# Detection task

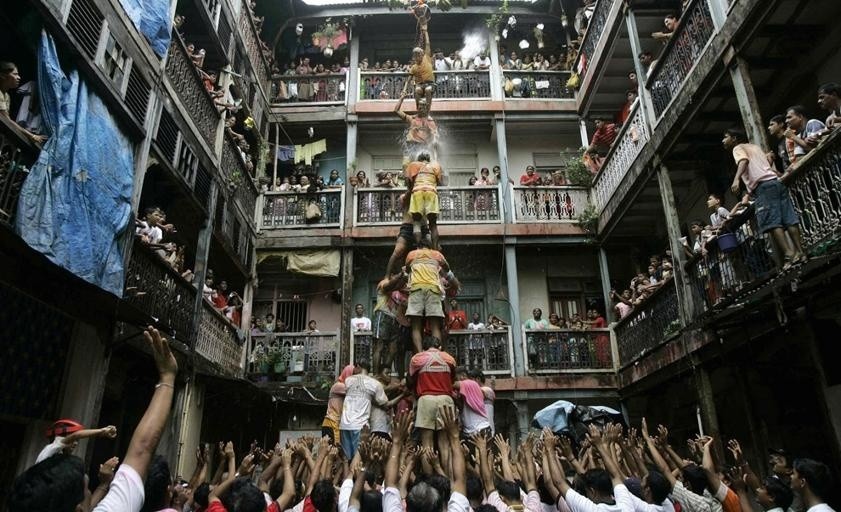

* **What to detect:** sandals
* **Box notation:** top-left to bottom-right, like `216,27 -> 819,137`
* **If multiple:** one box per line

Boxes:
779,252 -> 807,271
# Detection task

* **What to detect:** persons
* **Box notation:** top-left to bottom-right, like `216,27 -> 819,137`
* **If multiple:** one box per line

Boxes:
243,1 -> 416,108
467,1 -> 707,221
2,63 -> 50,148
174,12 -> 408,224
302,320 -> 321,372
2,324 -> 840,512
125,206 -> 242,341
609,86 -> 839,366
433,2 -> 609,98
370,1 -> 464,386
250,313 -> 292,372
324,304 -> 372,373
467,303 -> 614,380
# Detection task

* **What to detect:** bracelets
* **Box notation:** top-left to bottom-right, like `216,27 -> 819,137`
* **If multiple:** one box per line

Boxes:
360,467 -> 366,471
155,383 -> 174,389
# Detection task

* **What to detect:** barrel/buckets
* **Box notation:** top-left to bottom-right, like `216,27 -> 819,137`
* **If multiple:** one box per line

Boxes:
716,224 -> 738,253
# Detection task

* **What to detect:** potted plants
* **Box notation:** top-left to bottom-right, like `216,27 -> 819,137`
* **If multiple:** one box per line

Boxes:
348,157 -> 359,187
255,353 -> 269,371
533,25 -> 543,49
311,17 -> 349,57
269,347 -> 286,373
294,354 -> 304,371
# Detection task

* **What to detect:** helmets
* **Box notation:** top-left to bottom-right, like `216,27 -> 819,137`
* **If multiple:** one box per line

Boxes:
47,420 -> 83,437
